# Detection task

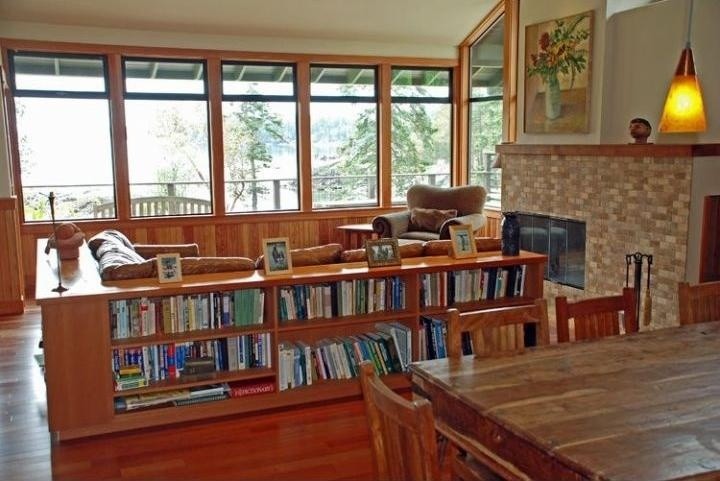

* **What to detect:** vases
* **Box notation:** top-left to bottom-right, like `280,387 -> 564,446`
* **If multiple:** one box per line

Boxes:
502,211 -> 520,256
546,74 -> 562,119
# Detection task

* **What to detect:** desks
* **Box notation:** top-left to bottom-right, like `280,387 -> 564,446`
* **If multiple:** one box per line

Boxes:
408,321 -> 720,481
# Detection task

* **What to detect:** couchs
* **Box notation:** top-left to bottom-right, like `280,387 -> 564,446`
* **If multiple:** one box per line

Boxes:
372,185 -> 486,239
88,228 -> 501,281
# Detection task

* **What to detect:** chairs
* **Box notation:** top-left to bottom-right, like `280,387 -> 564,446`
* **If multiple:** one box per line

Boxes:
679,282 -> 720,324
555,288 -> 637,343
441,298 -> 550,481
360,360 -> 440,481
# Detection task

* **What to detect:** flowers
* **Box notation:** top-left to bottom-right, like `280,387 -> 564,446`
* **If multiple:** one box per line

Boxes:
526,16 -> 590,89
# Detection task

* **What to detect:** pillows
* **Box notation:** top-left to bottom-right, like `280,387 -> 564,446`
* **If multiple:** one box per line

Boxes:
408,208 -> 458,232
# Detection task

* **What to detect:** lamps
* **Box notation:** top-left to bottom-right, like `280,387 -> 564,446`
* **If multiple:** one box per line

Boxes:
657,0 -> 707,134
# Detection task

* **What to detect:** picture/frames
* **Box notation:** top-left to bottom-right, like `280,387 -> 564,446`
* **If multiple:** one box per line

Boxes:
366,238 -> 401,268
156,252 -> 181,284
262,236 -> 292,275
524,9 -> 595,135
448,225 -> 478,259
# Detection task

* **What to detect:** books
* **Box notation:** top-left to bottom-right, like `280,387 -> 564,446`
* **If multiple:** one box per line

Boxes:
279,321 -> 413,391
109,288 -> 265,341
278,276 -> 406,321
115,379 -> 273,412
418,314 -> 475,362
418,264 -> 526,308
112,332 -> 272,391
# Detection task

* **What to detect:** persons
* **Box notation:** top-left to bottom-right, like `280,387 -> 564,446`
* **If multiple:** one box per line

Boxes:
628,118 -> 655,146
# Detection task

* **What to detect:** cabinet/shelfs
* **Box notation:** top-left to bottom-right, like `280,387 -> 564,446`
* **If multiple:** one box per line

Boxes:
35,237 -> 549,441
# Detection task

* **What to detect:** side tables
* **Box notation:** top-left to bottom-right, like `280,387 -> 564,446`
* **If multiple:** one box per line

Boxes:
336,223 -> 373,249
